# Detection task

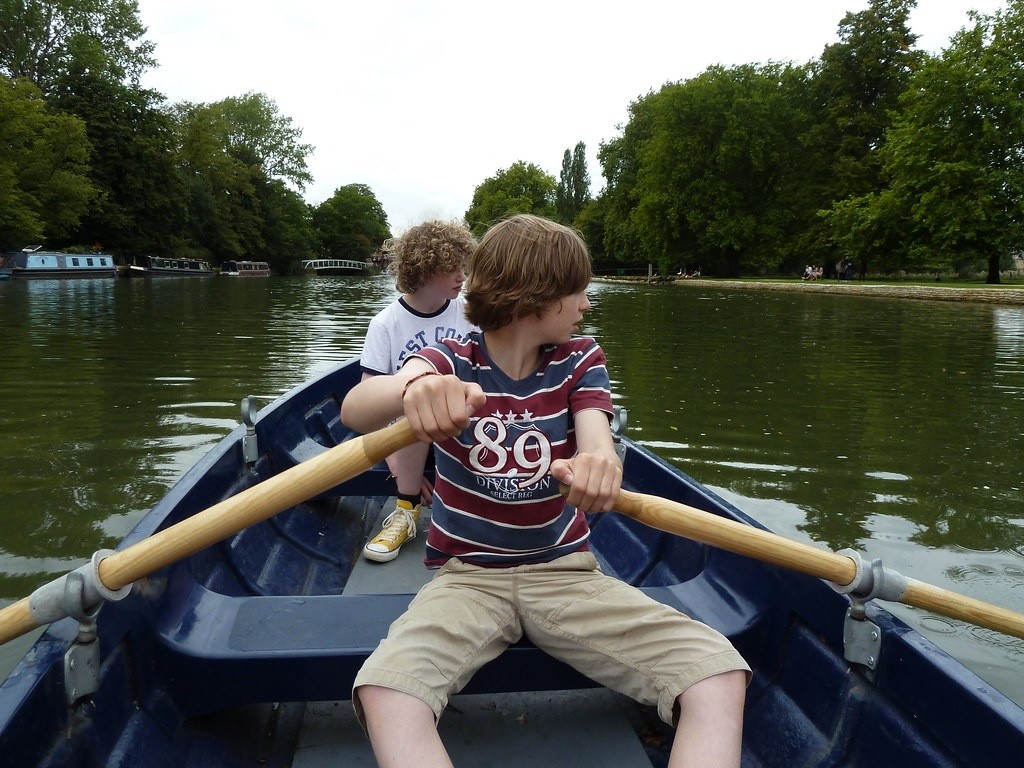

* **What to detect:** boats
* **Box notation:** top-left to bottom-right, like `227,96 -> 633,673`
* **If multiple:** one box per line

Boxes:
11,245 -> 117,277
216,260 -> 272,278
0,356 -> 1024,768
127,254 -> 213,276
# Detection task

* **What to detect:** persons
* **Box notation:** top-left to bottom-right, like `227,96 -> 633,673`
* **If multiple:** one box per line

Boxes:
676,268 -> 700,278
801,253 -> 868,281
341,213 -> 752,768
358,217 -> 478,563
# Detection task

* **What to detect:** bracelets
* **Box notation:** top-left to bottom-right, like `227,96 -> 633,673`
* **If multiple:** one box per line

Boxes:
402,371 -> 442,402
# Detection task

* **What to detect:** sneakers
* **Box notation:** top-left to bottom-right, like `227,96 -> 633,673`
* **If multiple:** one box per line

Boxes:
363,497 -> 423,562
